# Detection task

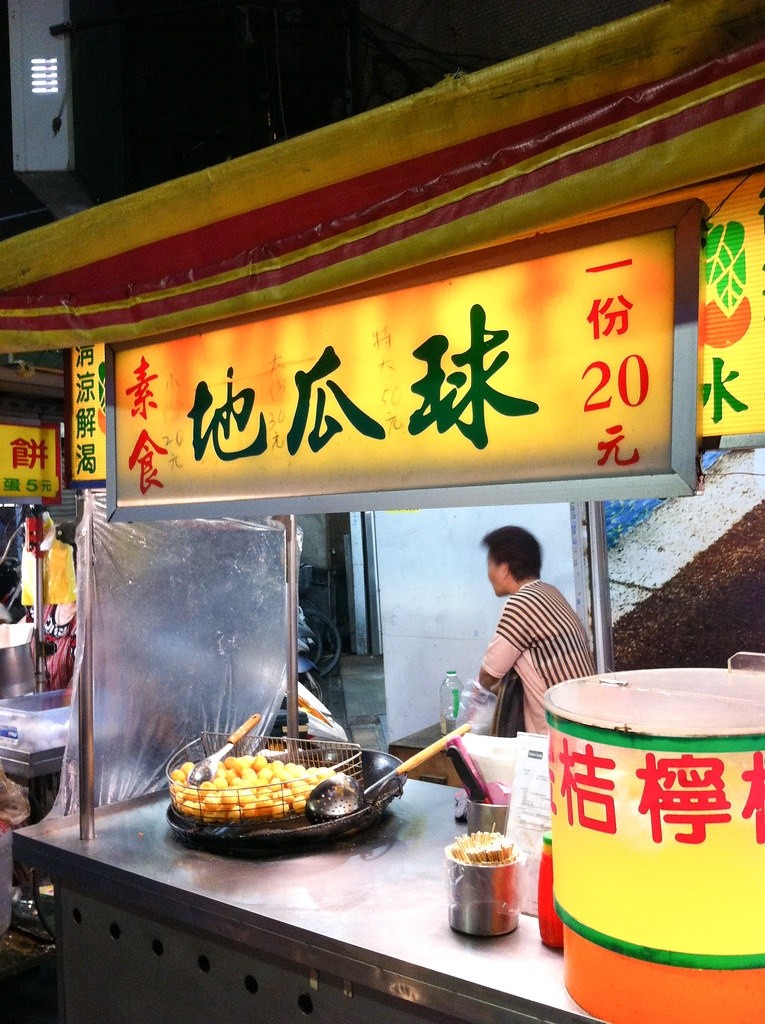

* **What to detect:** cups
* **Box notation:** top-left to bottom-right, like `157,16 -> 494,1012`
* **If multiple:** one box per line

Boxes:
443,847 -> 522,936
466,797 -> 508,836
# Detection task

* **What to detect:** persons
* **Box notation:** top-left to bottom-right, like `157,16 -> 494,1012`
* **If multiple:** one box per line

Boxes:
479,526 -> 596,735
0,564 -> 19,678
17,599 -> 76,708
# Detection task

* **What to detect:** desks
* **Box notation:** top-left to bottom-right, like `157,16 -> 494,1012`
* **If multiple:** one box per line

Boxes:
388,721 -> 461,787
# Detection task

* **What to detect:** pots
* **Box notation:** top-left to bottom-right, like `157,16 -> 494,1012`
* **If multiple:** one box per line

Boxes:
163,747 -> 407,854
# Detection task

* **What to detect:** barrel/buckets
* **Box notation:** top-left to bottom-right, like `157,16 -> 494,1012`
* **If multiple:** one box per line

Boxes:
0,643 -> 37,698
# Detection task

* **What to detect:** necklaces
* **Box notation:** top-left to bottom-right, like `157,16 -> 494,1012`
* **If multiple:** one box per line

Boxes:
55,607 -> 76,627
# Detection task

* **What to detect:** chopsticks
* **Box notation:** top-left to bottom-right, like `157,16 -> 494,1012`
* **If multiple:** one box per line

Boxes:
451,822 -> 516,864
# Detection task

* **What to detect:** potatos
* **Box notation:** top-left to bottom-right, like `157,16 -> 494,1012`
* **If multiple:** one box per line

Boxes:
170,755 -> 340,823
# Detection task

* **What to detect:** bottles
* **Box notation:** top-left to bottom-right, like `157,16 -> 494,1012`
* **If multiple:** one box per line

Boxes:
438,670 -> 464,736
536,831 -> 564,948
